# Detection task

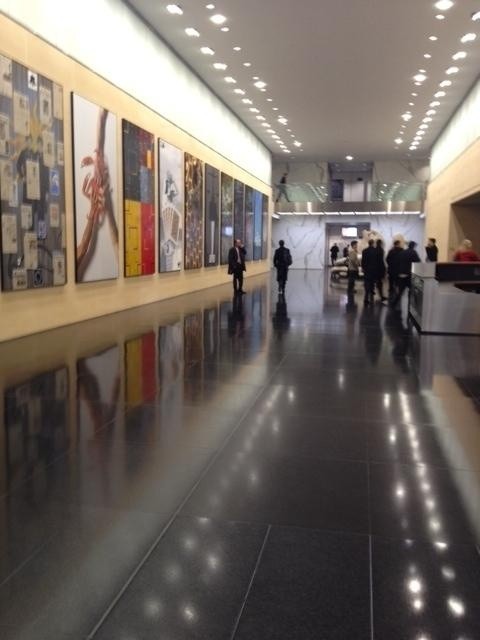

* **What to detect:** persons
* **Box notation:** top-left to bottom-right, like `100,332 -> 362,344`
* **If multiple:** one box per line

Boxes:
228,238 -> 247,296
453,239 -> 480,261
330,236 -> 438,307
274,239 -> 292,289
80,156 -> 118,249
276,172 -> 291,203
